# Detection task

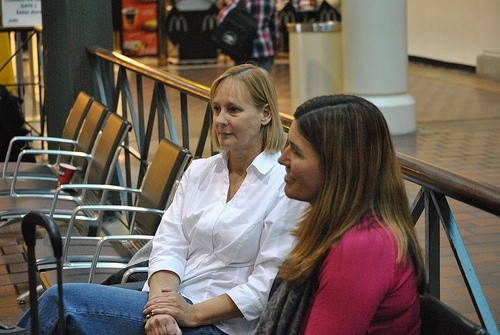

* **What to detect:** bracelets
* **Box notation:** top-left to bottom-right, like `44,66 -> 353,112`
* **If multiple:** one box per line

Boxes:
146,311 -> 156,320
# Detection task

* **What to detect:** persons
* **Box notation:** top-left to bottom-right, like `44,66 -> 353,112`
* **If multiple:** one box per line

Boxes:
217,0 -> 280,74
253,92 -> 423,335
18,61 -> 311,335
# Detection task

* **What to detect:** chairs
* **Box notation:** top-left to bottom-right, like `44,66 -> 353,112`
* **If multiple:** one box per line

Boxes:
0,91 -> 491,335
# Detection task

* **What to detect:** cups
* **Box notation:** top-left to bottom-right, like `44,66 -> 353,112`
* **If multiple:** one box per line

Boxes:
57,162 -> 77,186
